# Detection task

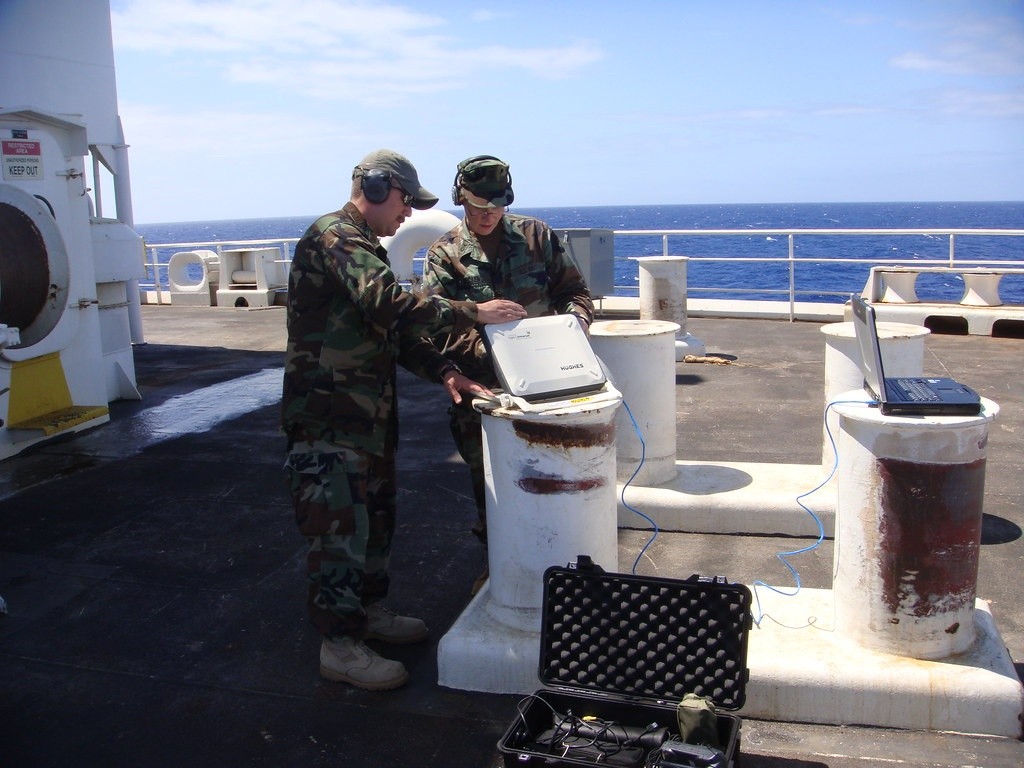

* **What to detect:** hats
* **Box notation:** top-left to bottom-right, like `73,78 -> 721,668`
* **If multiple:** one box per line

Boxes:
353,155 -> 439,210
457,157 -> 514,209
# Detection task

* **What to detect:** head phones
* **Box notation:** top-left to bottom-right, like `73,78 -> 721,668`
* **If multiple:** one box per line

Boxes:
361,168 -> 393,204
451,155 -> 512,206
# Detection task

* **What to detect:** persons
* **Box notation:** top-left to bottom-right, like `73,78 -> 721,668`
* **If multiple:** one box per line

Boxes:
279,149 -> 528,692
422,154 -> 595,597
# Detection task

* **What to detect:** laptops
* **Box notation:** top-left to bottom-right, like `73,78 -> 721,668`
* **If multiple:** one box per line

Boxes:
850,293 -> 983,417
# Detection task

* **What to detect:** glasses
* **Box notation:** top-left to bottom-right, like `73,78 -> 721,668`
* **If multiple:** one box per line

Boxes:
391,186 -> 416,206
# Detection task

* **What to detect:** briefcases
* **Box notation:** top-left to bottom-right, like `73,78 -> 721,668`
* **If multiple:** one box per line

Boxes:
496,554 -> 752,768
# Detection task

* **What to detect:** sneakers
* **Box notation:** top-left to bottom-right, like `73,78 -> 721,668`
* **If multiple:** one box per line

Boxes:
319,635 -> 408,691
362,609 -> 429,644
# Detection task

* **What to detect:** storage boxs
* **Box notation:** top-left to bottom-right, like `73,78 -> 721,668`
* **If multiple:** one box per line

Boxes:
495,555 -> 754,768
